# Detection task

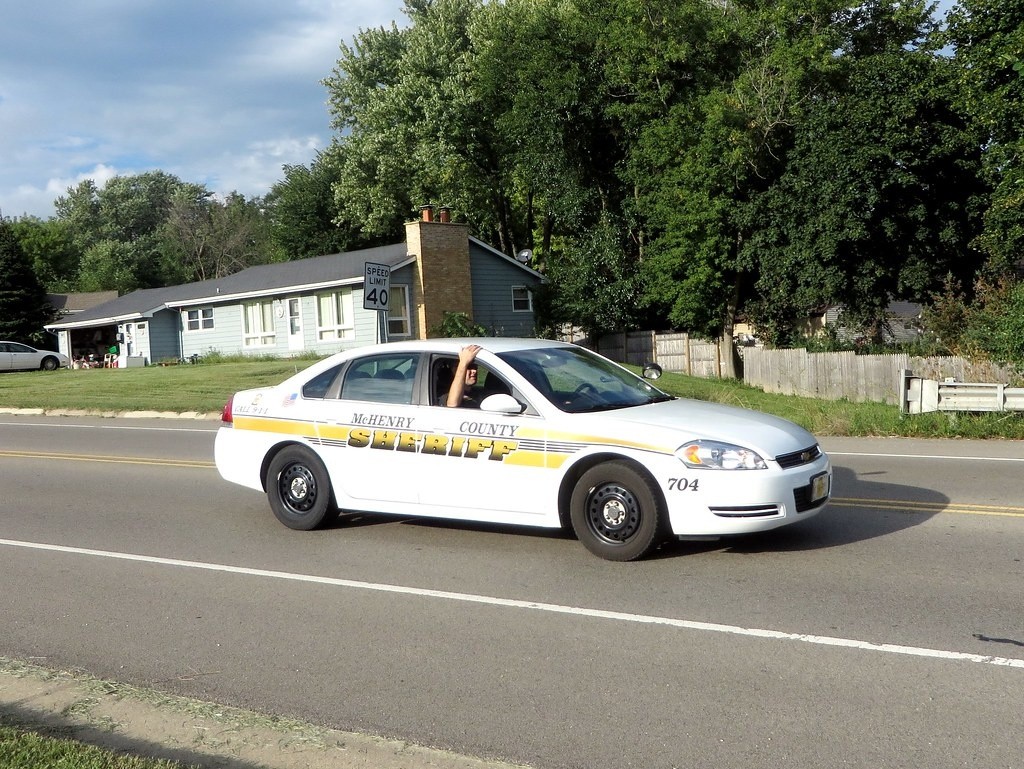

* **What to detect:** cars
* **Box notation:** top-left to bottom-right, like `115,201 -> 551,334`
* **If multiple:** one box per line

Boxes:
0,340 -> 69,371
214,338 -> 834,562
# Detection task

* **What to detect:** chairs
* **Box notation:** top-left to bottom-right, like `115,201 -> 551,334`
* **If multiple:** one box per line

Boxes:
483,371 -> 511,397
103,354 -> 113,368
373,369 -> 410,404
344,371 -> 383,403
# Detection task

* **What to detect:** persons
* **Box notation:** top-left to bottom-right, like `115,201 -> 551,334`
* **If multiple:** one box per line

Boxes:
441,344 -> 483,408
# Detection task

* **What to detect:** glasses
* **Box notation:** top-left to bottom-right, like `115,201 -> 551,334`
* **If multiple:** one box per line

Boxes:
453,363 -> 478,370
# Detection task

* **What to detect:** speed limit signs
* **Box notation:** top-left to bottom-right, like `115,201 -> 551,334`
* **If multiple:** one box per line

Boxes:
362,262 -> 390,311
